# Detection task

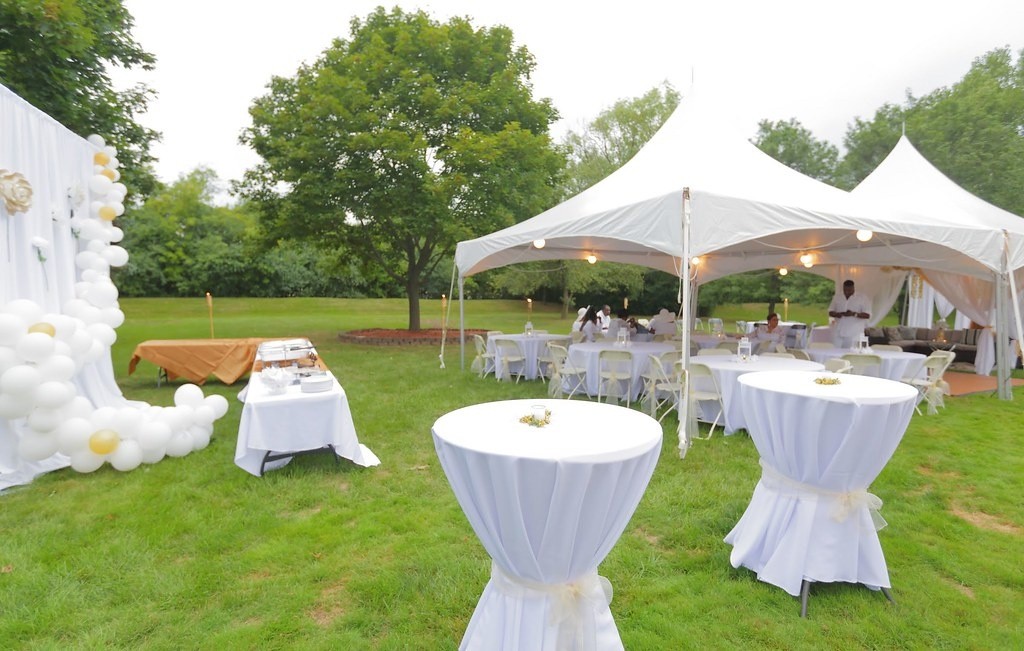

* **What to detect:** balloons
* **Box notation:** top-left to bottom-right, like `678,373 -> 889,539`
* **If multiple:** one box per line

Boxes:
0,134 -> 229,472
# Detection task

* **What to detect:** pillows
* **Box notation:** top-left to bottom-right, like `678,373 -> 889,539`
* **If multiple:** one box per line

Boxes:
864,327 -> 982,345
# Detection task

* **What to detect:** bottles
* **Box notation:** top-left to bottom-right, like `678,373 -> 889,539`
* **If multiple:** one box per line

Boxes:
525,322 -> 533,336
738,337 -> 751,358
617,328 -> 630,345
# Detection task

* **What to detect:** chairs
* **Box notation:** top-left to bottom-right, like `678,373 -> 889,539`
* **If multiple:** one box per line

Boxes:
472,320 -> 956,440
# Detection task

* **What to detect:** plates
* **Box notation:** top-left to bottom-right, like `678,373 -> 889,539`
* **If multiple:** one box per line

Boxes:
300,375 -> 333,393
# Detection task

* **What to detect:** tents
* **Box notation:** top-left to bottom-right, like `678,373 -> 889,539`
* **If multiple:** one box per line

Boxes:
456,77 -> 1024,454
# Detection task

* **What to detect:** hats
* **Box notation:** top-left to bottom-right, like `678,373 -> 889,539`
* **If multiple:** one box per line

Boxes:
654,309 -> 676,323
576,305 -> 591,322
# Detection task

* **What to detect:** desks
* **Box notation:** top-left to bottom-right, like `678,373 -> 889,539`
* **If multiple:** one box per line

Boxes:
723,371 -> 919,619
811,326 -> 834,342
634,331 -> 720,350
801,346 -> 926,382
673,356 -> 827,436
234,338 -> 380,478
668,334 -> 776,356
749,321 -> 808,348
564,341 -> 676,401
127,338 -> 303,388
488,335 -> 572,381
433,400 -> 663,651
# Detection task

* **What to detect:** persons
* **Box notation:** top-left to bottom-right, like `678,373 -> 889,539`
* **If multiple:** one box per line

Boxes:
649,309 -> 677,335
736,313 -> 786,352
828,280 -> 870,350
606,308 -> 638,340
579,305 -> 603,342
596,304 -> 611,329
572,308 -> 586,331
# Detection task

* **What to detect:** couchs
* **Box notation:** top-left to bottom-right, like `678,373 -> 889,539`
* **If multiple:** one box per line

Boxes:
860,326 -> 999,368
